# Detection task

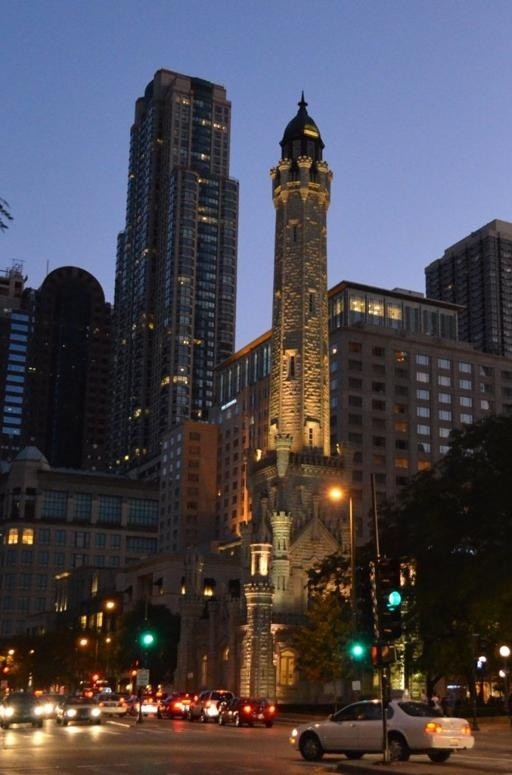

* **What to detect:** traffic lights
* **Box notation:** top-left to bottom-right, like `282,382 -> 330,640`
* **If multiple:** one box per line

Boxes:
371,645 -> 396,668
353,642 -> 364,666
135,625 -> 141,668
142,624 -> 154,668
356,560 -> 378,646
378,557 -> 402,640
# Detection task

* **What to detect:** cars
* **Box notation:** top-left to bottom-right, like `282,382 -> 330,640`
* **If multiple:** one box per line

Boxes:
288,699 -> 474,763
1,689 -> 276,729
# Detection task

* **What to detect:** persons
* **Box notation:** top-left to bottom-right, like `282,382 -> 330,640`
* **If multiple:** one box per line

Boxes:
420,687 -> 427,705
401,687 -> 411,701
428,696 -> 444,716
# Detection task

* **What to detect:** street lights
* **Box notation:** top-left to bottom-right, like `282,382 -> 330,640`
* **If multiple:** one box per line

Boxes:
329,487 -> 359,705
499,645 -> 511,712
